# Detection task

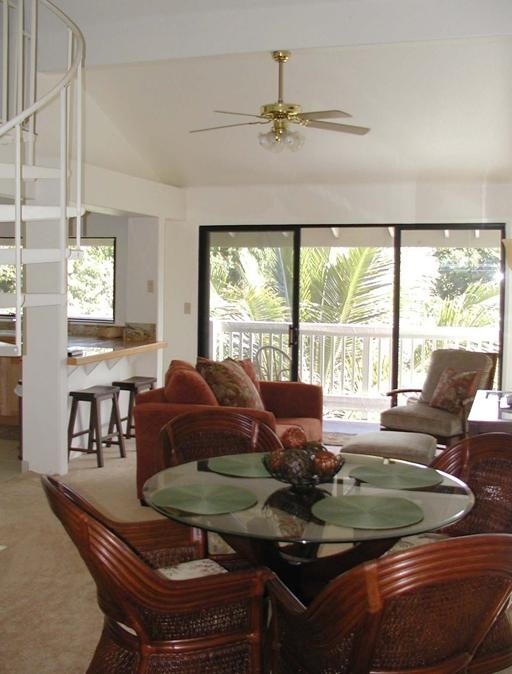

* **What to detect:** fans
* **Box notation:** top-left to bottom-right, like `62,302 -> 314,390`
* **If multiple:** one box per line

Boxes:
188,50 -> 375,138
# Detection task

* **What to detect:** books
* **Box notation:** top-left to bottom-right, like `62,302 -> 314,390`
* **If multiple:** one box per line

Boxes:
69,349 -> 84,357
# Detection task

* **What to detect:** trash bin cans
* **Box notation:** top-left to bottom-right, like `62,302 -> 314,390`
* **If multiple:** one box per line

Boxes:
14,379 -> 22,461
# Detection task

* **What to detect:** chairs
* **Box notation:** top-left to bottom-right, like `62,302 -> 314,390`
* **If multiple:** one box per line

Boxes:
377,348 -> 499,458
158,410 -> 284,469
254,345 -> 303,382
258,533 -> 512,674
430,432 -> 511,535
41,472 -> 271,672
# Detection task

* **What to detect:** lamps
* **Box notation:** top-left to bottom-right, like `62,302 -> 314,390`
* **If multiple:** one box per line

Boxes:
255,119 -> 304,151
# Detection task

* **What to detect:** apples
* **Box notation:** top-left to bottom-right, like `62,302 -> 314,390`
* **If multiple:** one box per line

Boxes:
270,428 -> 337,480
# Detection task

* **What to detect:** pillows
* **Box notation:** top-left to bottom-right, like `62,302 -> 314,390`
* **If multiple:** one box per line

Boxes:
426,364 -> 476,415
164,354 -> 266,410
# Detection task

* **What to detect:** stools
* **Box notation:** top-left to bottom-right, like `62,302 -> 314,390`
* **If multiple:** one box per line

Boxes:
103,377 -> 157,445
64,384 -> 127,468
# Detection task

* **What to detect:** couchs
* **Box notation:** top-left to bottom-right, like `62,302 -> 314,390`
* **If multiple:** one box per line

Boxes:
132,380 -> 325,511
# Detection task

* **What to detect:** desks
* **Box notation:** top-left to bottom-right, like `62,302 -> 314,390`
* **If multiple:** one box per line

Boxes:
143,448 -> 476,608
466,387 -> 512,440
